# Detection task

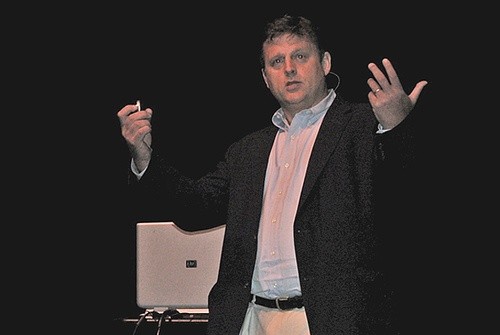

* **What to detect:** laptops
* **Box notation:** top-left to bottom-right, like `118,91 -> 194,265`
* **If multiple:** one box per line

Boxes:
137,221 -> 227,319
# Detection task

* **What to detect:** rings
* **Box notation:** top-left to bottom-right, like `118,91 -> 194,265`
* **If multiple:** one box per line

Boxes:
374,88 -> 381,94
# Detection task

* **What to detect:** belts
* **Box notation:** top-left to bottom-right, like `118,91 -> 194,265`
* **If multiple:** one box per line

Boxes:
249,294 -> 304,311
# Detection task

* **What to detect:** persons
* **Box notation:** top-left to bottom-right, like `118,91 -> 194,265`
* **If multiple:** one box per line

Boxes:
117,13 -> 443,335
